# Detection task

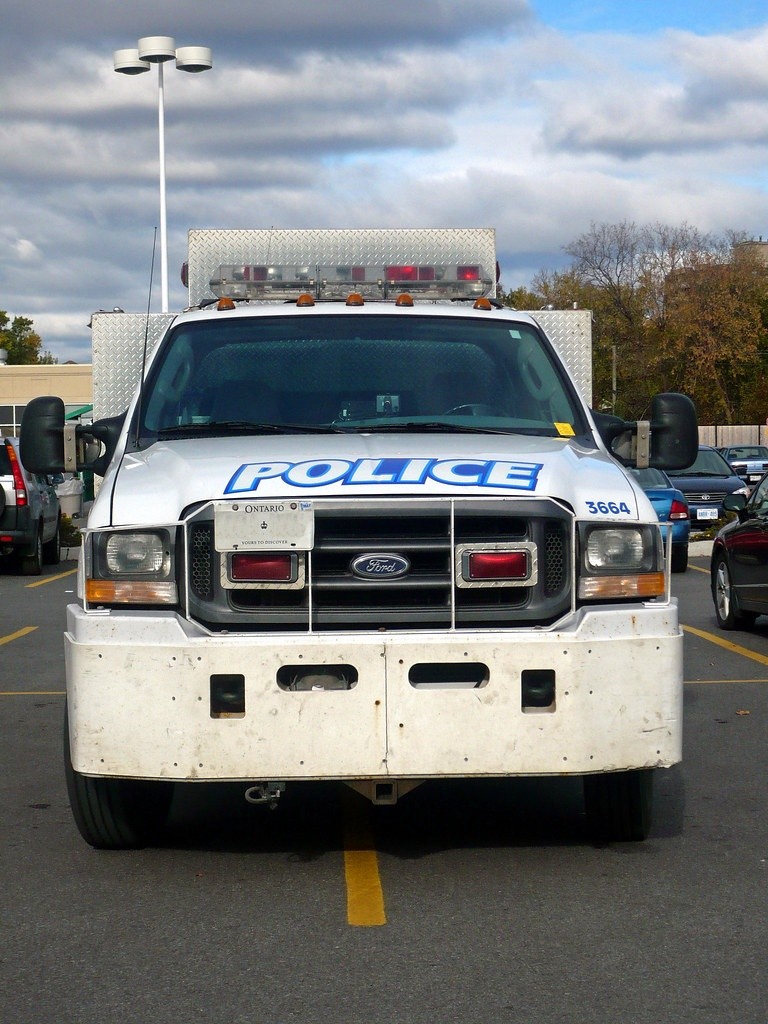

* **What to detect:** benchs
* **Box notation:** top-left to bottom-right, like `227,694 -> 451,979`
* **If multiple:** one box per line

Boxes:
278,386 -> 420,422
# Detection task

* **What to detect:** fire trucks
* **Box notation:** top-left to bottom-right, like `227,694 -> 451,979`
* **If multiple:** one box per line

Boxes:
20,223 -> 707,847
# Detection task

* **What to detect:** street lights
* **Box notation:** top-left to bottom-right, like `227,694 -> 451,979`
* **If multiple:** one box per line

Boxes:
110,34 -> 211,314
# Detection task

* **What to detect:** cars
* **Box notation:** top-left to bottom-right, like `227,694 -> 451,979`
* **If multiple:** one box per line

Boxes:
709,466 -> 768,629
661,444 -> 752,528
720,445 -> 768,483
628,465 -> 690,573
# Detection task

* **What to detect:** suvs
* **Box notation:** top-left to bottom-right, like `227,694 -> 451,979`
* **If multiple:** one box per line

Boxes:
0,436 -> 65,577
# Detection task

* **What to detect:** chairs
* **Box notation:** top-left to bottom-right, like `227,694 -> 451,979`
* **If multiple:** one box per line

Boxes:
424,371 -> 472,419
206,378 -> 280,424
